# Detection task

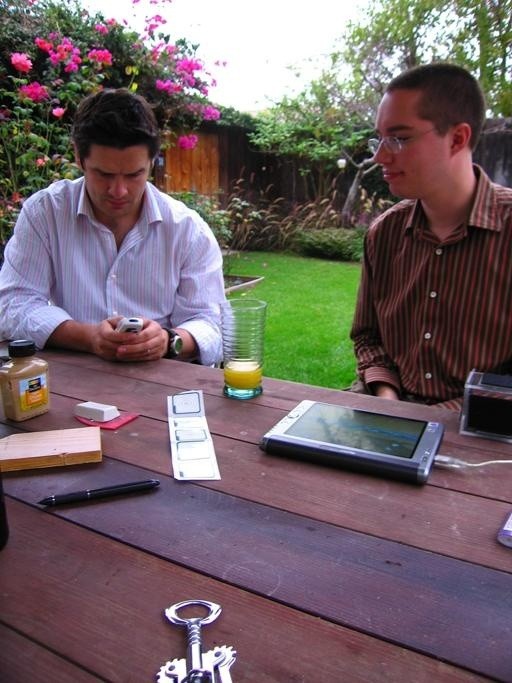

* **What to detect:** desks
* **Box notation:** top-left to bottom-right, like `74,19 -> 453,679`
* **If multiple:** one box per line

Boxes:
0,340 -> 512,683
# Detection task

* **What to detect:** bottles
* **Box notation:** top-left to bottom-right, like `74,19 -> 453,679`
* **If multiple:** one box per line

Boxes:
0,339 -> 51,423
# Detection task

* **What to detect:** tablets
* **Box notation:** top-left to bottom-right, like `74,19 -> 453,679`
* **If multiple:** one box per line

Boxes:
256,399 -> 445,483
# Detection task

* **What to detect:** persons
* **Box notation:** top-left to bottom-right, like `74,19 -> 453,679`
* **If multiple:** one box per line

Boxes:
350,63 -> 512,410
0,89 -> 235,364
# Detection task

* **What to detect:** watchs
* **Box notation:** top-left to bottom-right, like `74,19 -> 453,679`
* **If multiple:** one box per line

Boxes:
163,327 -> 182,359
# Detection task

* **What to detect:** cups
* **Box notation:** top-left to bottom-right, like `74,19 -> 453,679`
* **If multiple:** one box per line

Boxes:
220,299 -> 268,400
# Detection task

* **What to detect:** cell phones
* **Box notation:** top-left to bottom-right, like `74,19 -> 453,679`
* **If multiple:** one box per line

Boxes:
116,316 -> 143,332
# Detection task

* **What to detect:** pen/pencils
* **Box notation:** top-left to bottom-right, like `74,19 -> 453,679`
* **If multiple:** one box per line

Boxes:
36,479 -> 160,506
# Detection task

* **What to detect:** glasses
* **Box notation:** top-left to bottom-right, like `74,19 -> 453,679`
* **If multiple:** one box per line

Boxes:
365,123 -> 455,157
